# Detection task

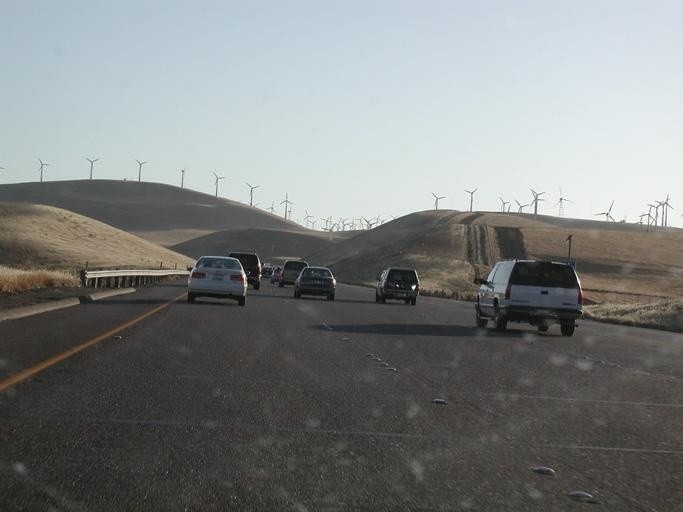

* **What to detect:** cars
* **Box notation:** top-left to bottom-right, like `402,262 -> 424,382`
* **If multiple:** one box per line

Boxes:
376,268 -> 420,305
187,252 -> 337,306
474,259 -> 583,336
